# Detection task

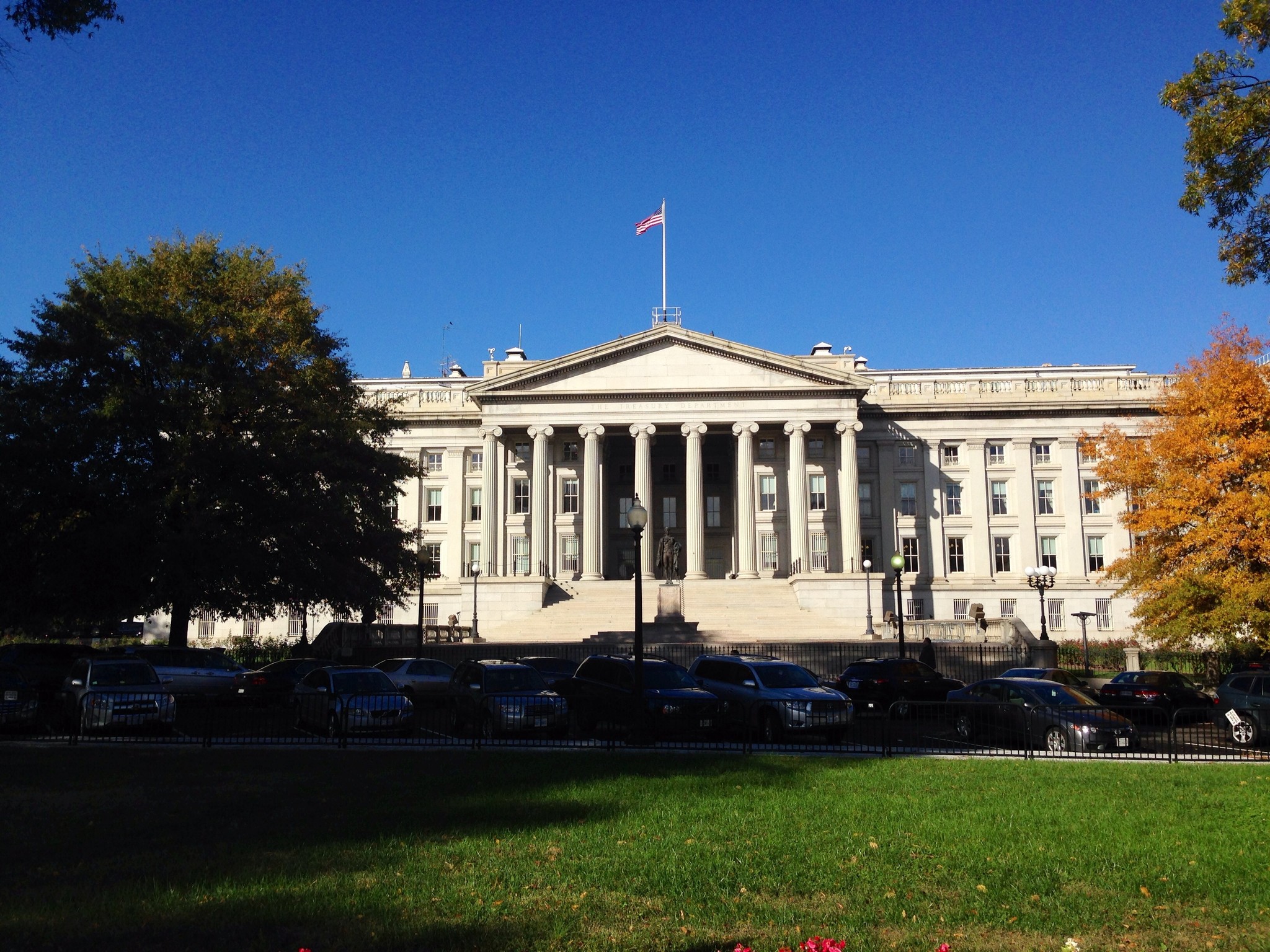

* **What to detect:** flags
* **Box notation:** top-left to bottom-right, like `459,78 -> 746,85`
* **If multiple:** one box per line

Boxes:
634,203 -> 664,237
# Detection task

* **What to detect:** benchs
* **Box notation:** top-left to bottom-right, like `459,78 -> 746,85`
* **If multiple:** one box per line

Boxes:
980,685 -> 1005,697
1123,677 -> 1146,684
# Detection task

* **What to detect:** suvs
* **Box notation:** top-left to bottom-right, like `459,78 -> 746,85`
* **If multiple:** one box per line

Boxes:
567,652 -> 721,739
126,645 -> 257,698
684,653 -> 855,745
1210,670 -> 1270,748
59,652 -> 177,731
834,657 -> 966,722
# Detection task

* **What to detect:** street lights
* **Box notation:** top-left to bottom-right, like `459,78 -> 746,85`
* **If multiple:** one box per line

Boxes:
415,546 -> 431,657
628,493 -> 649,745
471,559 -> 481,637
1025,565 -> 1057,640
862,558 -> 875,634
890,548 -> 906,658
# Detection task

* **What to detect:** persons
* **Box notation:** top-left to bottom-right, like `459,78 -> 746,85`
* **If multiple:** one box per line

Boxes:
656,527 -> 677,583
730,650 -> 739,656
920,637 -> 937,672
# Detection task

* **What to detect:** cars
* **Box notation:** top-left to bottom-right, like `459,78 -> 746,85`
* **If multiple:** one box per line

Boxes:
234,657 -> 345,709
1000,666 -> 1100,703
945,675 -> 1141,756
513,656 -> 581,691
0,658 -> 43,730
1102,670 -> 1216,728
444,660 -> 568,741
289,664 -> 416,737
372,658 -> 456,706
